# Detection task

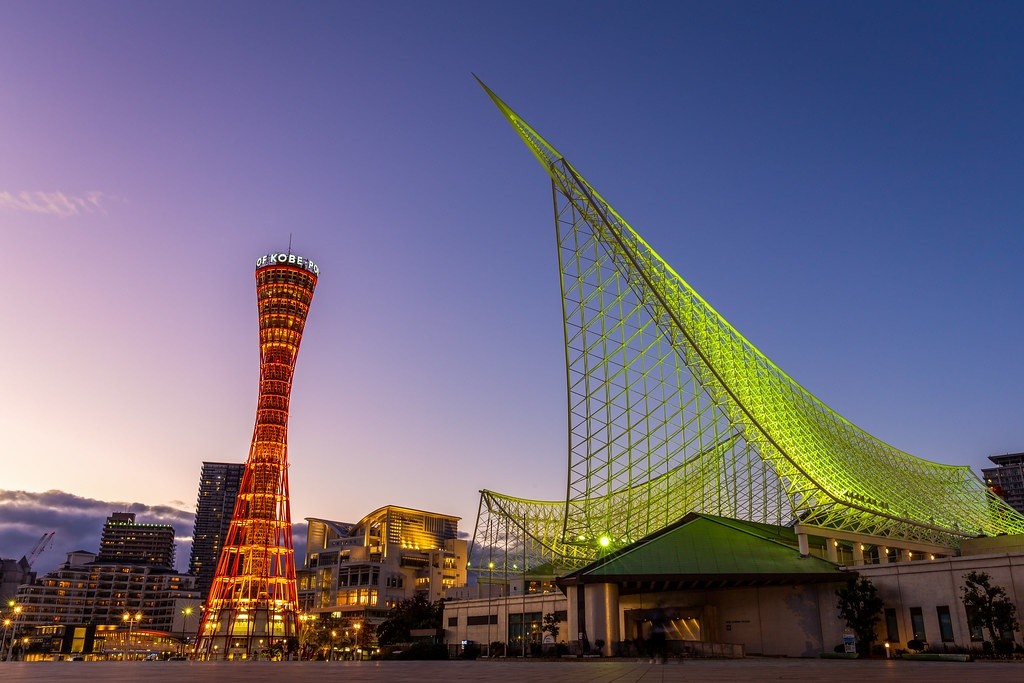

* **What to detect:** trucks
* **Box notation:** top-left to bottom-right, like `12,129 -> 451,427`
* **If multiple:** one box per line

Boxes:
146,654 -> 163,661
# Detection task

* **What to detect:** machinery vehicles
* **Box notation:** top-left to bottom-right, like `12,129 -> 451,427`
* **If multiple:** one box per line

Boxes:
27,532 -> 56,567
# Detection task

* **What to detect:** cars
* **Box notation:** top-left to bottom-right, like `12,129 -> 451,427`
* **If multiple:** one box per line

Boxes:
67,656 -> 84,662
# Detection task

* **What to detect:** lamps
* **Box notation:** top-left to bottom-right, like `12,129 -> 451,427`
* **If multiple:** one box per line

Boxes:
885,642 -> 891,658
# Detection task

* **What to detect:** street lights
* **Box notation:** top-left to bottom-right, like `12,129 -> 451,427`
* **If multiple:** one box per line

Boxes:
215,645 -> 219,660
22,638 -> 29,661
283,639 -> 287,660
258,639 -> 264,661
181,608 -> 191,643
0,607 -> 21,661
330,631 -> 336,662
488,562 -> 495,658
353,623 -> 361,661
121,612 -> 143,632
299,615 -> 319,661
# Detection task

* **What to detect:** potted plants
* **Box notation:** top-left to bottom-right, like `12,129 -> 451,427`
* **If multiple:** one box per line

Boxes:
645,631 -> 666,664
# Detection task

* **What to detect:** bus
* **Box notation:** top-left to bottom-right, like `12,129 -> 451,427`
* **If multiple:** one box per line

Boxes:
168,657 -> 186,662
